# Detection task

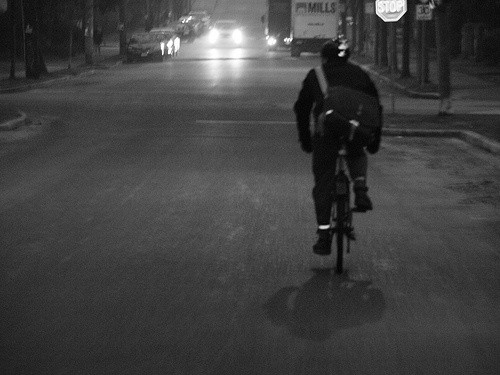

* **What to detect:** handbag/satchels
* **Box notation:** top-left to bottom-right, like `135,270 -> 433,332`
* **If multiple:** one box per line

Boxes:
313,64 -> 380,157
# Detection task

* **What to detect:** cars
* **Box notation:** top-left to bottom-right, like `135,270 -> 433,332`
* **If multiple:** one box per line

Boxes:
129,7 -> 211,66
206,18 -> 244,48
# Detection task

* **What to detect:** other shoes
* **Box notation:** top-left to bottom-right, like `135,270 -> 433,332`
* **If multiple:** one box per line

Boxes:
313,228 -> 331,254
355,191 -> 372,210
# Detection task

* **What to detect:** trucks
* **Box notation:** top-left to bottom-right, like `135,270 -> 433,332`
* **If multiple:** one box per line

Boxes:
260,0 -> 294,55
287,1 -> 344,59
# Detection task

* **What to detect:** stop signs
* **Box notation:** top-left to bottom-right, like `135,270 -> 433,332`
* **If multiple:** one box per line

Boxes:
376,0 -> 408,23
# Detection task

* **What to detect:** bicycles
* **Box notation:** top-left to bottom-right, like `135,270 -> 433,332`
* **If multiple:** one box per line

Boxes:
327,138 -> 362,276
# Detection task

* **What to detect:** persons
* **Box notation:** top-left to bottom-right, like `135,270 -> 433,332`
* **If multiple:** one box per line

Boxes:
294,34 -> 384,255
